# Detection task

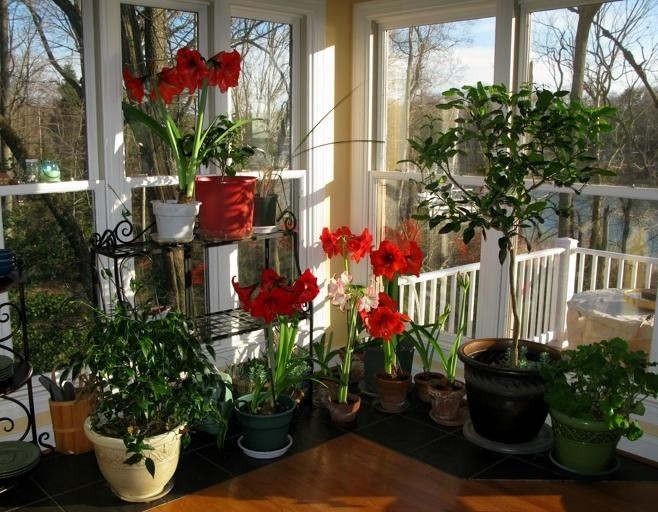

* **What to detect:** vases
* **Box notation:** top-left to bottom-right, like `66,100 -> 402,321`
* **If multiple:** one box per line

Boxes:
363,345 -> 413,394
152,199 -> 199,244
340,346 -> 366,380
372,373 -> 411,412
232,393 -> 297,460
323,394 -> 361,422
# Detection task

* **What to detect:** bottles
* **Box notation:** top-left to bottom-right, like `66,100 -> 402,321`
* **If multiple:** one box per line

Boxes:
24,159 -> 40,183
41,160 -> 61,181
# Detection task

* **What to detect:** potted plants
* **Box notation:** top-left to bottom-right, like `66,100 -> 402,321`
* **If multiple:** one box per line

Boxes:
404,83 -> 621,456
403,306 -> 451,402
538,337 -> 657,475
180,115 -> 256,241
253,119 -> 288,226
58,299 -> 236,504
427,272 -> 467,425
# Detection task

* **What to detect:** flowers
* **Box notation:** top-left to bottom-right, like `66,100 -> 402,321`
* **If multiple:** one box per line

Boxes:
229,268 -> 330,414
122,46 -> 242,202
360,290 -> 411,375
369,240 -> 423,351
321,225 -> 373,352
321,271 -> 381,404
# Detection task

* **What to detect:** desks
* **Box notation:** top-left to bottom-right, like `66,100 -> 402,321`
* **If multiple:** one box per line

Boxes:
566,289 -> 655,373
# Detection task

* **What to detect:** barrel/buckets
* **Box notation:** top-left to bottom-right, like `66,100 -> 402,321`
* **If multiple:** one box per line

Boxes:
49,386 -> 97,454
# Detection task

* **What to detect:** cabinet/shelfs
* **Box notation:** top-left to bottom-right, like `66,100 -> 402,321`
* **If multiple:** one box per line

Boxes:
91,211 -> 314,398
1,249 -> 55,479
